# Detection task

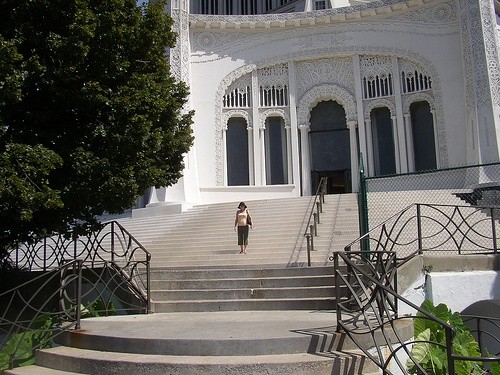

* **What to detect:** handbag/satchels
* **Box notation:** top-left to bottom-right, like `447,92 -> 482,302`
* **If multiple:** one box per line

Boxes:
246,210 -> 251,224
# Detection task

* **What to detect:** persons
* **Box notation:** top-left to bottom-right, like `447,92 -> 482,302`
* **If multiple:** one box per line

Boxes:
234,202 -> 252,254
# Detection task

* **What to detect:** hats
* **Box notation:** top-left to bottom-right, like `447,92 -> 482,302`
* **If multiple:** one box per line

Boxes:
237,202 -> 247,208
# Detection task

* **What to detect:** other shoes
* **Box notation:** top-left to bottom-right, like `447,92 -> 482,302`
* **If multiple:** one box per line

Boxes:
240,250 -> 247,254
